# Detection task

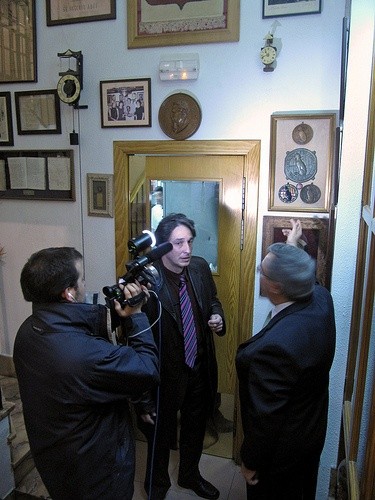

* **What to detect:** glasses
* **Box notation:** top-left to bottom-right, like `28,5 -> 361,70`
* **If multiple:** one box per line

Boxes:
256,264 -> 276,282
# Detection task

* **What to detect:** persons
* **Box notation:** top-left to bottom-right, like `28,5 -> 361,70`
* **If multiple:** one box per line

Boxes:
13,247 -> 159,500
108,93 -> 144,121
234,219 -> 336,500
151,185 -> 164,227
136,213 -> 227,500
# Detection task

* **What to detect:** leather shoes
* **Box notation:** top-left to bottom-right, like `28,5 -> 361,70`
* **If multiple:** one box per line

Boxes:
178,475 -> 221,500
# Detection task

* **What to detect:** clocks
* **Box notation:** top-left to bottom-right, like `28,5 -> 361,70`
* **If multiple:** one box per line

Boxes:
260,31 -> 277,72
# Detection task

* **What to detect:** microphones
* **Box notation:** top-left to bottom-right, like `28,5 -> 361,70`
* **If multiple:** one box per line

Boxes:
136,242 -> 174,267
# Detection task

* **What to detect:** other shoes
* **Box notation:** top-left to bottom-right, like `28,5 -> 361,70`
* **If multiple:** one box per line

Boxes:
205,416 -> 234,433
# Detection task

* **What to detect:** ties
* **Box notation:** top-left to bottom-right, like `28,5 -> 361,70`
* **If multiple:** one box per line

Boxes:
178,275 -> 198,368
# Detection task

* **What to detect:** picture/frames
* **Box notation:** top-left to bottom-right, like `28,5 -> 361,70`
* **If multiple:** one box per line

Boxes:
0,0 -> 39,84
86,172 -> 114,218
0,90 -> 14,147
46,0 -> 116,27
262,0 -> 321,18
14,89 -> 62,135
127,0 -> 241,48
260,216 -> 336,293
267,112 -> 337,213
99,78 -> 152,128
